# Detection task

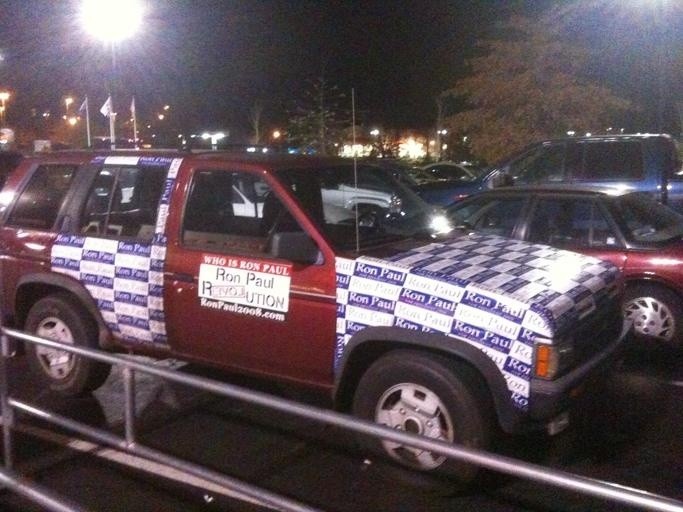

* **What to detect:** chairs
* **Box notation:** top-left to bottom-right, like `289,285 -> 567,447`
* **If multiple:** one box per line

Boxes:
86,171 -> 327,241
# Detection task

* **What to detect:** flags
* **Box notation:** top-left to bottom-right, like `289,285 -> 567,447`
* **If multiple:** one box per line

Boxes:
130,96 -> 135,121
98,96 -> 112,118
78,99 -> 86,113
